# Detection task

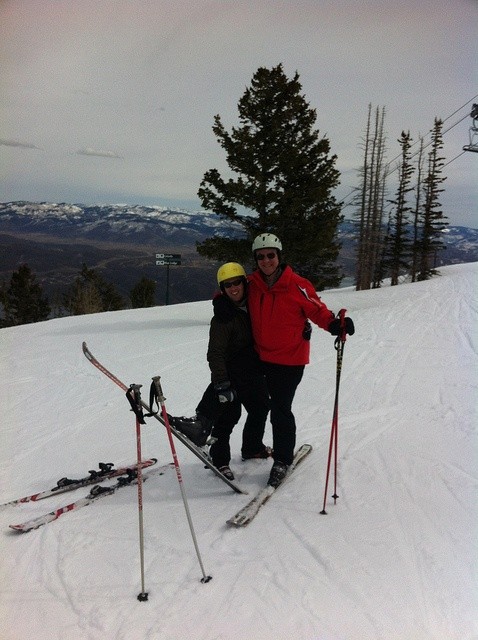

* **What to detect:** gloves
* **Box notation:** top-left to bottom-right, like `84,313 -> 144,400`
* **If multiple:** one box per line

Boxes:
303,323 -> 312,340
214,381 -> 236,403
330,317 -> 354,336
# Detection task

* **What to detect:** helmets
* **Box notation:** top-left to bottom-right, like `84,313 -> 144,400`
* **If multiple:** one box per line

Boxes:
251,233 -> 283,277
217,263 -> 248,302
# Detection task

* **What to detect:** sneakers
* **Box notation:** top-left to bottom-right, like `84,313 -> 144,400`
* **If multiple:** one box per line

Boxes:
241,447 -> 272,459
270,458 -> 288,479
173,415 -> 210,446
211,457 -> 234,480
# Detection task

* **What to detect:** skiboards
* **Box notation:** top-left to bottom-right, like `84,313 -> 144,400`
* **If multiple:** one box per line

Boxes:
0,457 -> 178,533
81,342 -> 313,526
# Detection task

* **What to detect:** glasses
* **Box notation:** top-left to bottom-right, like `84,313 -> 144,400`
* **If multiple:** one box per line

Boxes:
221,278 -> 242,289
256,253 -> 276,261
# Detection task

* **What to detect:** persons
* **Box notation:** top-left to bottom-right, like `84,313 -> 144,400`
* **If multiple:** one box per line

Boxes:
207,261 -> 275,481
160,232 -> 356,488
